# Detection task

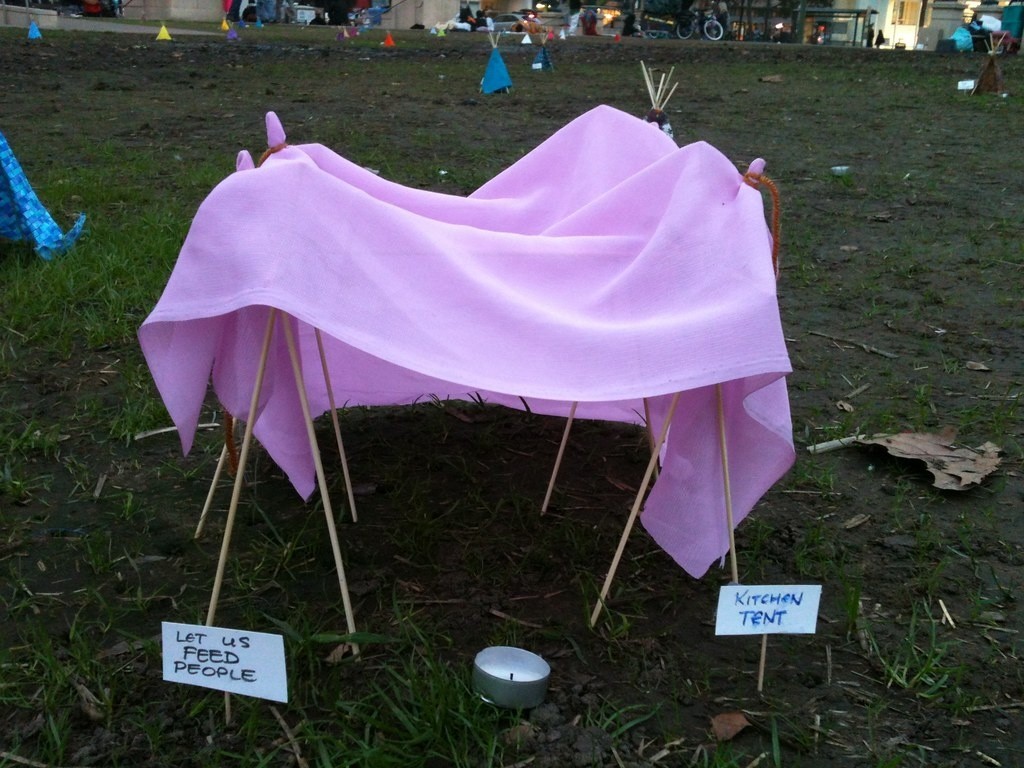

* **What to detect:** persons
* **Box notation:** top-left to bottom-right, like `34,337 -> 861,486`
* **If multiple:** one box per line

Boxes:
690,0 -> 729,40
460,8 -> 488,32
866,25 -> 885,49
310,9 -> 326,25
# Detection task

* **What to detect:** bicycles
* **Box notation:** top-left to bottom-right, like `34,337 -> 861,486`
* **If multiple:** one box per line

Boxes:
676,8 -> 725,41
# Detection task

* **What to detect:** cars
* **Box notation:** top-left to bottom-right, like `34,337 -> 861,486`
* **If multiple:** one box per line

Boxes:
492,13 -> 541,33
